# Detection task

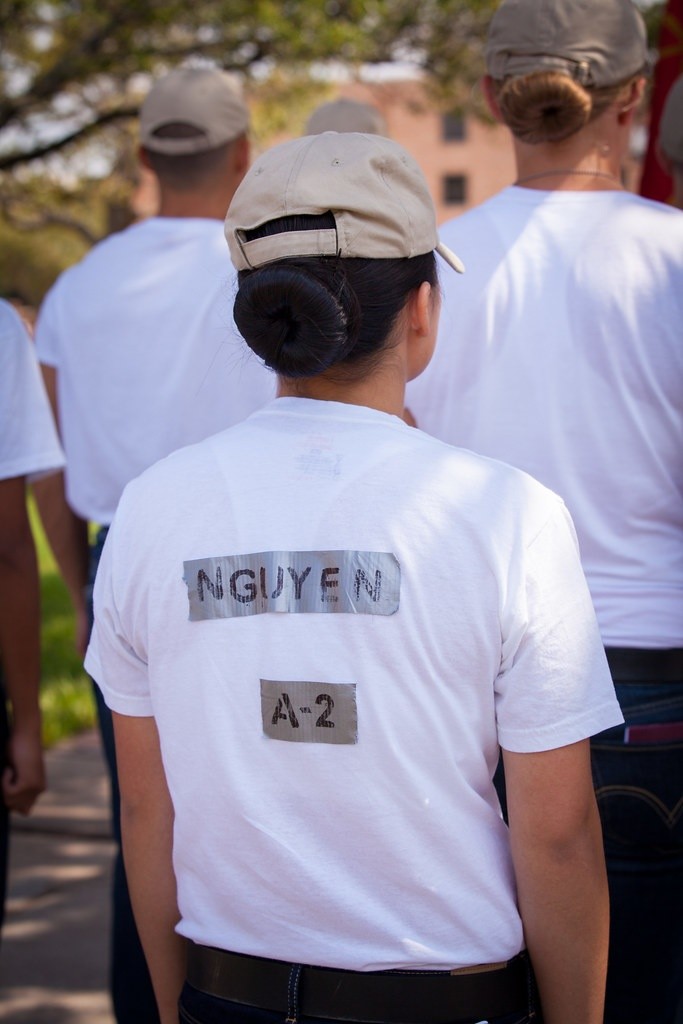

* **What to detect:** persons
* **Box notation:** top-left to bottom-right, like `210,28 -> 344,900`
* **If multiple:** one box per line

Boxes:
81,130 -> 627,1024
304,98 -> 386,136
0,296 -> 64,940
34,60 -> 279,850
403,0 -> 683,1024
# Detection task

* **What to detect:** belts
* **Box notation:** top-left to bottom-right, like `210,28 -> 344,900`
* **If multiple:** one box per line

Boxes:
604,647 -> 683,682
185,939 -> 540,1024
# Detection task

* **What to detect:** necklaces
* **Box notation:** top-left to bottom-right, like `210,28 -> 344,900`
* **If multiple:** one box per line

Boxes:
513,168 -> 616,184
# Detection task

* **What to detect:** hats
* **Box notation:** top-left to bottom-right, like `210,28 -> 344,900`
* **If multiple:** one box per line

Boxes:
304,95 -> 384,135
660,75 -> 683,160
483,0 -> 647,84
224,130 -> 465,273
139,69 -> 249,152
